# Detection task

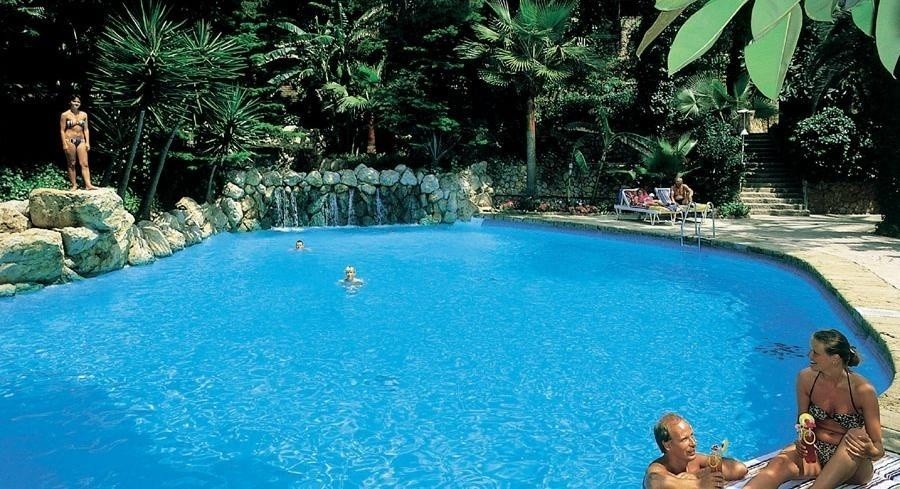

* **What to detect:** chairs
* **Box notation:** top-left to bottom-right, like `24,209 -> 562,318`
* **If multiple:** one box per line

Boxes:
615,189 -> 712,226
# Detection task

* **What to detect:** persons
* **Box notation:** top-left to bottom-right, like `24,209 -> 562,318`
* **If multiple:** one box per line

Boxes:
634,189 -> 673,215
59,93 -> 98,190
341,267 -> 364,290
743,331 -> 883,489
289,240 -> 308,251
671,178 -> 695,207
643,414 -> 747,489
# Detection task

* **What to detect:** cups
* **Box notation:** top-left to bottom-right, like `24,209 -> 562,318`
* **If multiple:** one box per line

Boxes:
710,445 -> 724,487
803,437 -> 817,464
